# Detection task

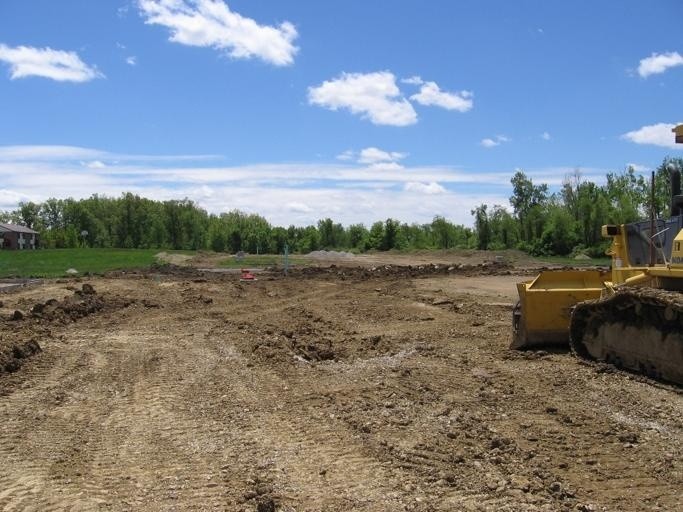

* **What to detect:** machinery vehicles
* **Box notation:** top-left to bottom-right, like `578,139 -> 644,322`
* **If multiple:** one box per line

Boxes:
509,123 -> 683,396
